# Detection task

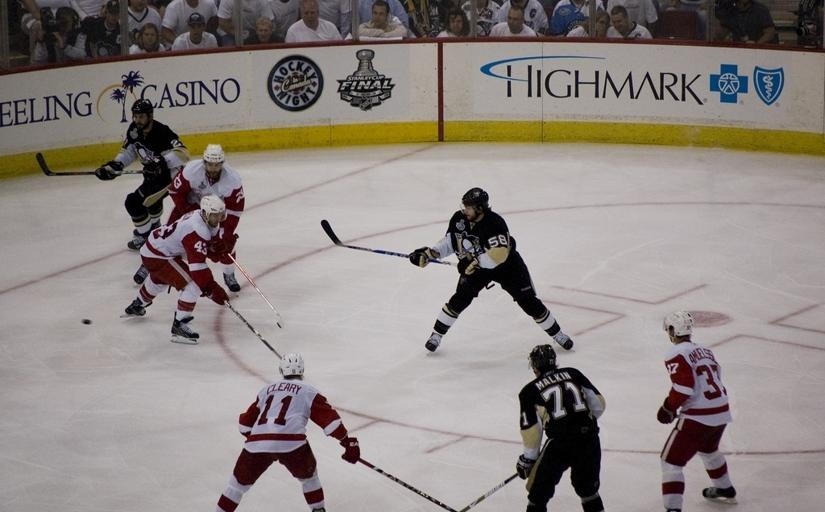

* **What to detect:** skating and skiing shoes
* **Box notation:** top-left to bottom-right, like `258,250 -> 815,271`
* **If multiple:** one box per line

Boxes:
124,297 -> 151,317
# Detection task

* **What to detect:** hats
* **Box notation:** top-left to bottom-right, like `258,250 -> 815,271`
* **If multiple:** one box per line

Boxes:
106,0 -> 120,14
187,13 -> 205,27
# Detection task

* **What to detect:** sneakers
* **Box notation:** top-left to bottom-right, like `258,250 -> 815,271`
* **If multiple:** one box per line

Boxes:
701,487 -> 737,499
126,236 -> 145,250
170,312 -> 199,339
551,329 -> 573,350
222,272 -> 240,293
424,330 -> 442,353
132,263 -> 149,285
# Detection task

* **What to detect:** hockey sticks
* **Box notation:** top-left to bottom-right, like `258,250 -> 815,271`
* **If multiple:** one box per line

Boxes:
358,455 -> 454,512
36,151 -> 158,178
230,252 -> 284,330
322,217 -> 473,271
455,470 -> 520,510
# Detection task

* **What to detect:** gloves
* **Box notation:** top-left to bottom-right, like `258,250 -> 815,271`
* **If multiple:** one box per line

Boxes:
94,160 -> 123,181
408,246 -> 440,268
456,254 -> 479,278
200,282 -> 229,305
656,396 -> 679,424
514,454 -> 536,481
209,239 -> 227,255
339,436 -> 361,464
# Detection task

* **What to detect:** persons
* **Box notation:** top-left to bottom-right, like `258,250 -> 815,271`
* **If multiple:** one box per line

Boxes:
97,98 -> 193,250
406,187 -> 575,353
513,340 -> 609,510
132,143 -> 247,292
653,308 -> 738,510
213,348 -> 361,511
1,1 -> 824,78
123,193 -> 230,339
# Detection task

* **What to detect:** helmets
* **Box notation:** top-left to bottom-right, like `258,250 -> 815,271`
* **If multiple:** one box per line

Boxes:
199,195 -> 227,226
278,351 -> 305,378
130,98 -> 153,130
459,188 -> 489,216
203,144 -> 224,172
662,309 -> 694,343
527,343 -> 557,374
